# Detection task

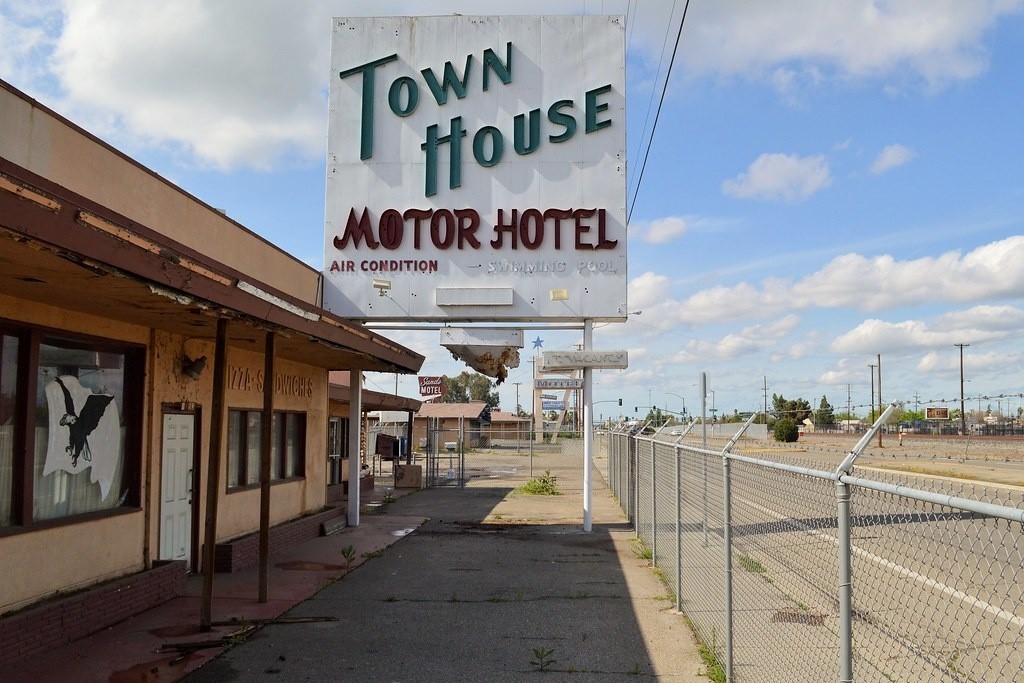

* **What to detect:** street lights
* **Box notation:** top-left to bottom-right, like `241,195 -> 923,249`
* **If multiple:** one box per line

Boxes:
692,384 -> 714,437
666,393 -> 685,431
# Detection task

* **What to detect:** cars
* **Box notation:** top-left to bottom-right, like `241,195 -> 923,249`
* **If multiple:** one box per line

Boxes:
670,429 -> 682,436
597,431 -> 604,435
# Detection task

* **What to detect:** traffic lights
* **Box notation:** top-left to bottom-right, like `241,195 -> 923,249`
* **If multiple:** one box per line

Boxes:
635,407 -> 638,412
653,406 -> 656,411
619,399 -> 622,406
